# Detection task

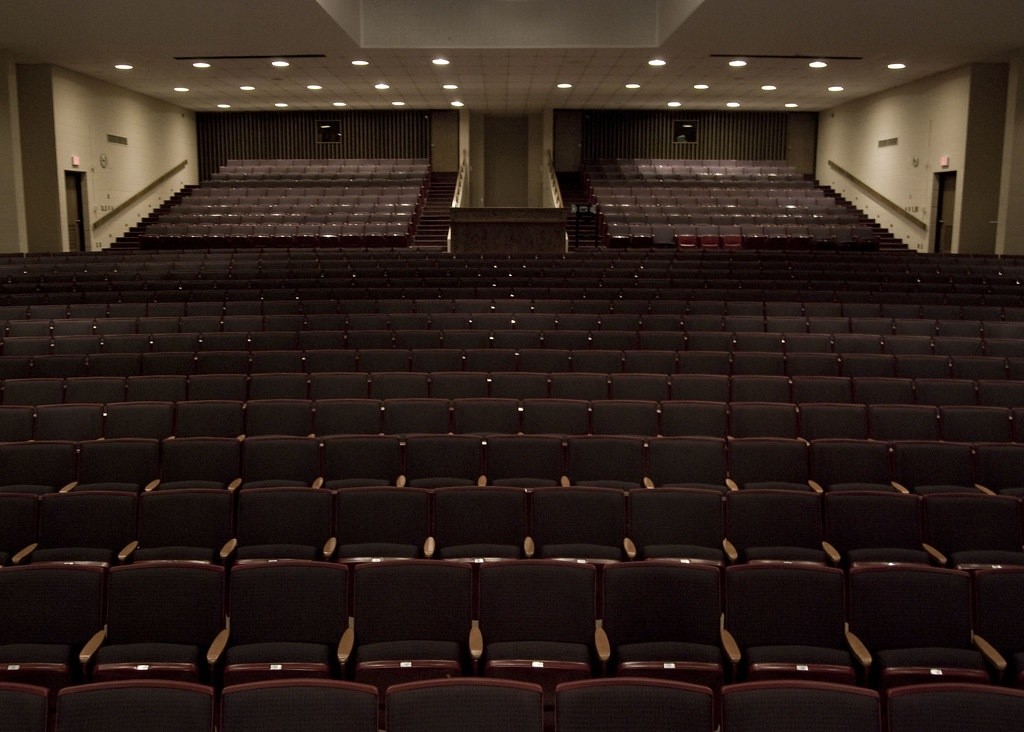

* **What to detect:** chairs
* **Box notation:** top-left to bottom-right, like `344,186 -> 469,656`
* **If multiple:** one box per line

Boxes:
0,158 -> 1024,732
